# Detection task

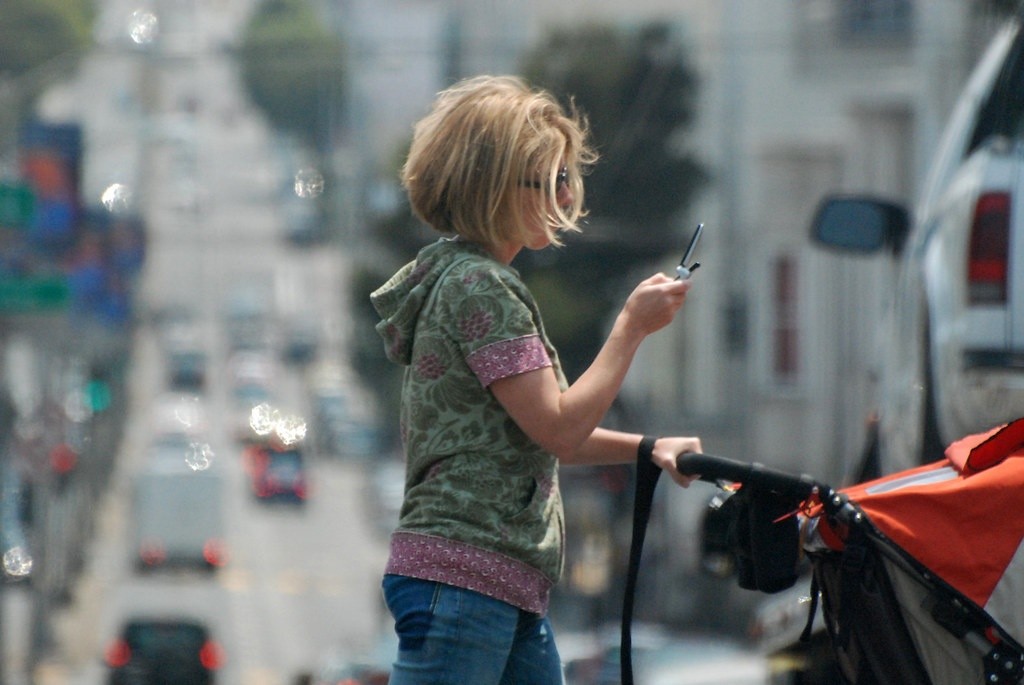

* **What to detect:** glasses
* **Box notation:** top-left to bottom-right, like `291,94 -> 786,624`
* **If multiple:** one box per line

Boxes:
516,168 -> 570,194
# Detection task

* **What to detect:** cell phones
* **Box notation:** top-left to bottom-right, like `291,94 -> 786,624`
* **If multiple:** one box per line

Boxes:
674,222 -> 704,282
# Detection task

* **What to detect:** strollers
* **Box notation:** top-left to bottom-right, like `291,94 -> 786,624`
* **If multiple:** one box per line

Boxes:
676,415 -> 1024,685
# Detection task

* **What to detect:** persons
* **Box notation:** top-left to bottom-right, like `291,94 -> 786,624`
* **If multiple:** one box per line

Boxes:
368,73 -> 704,684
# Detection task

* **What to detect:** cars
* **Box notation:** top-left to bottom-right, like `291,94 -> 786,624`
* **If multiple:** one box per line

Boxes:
254,445 -> 308,501
102,618 -> 221,685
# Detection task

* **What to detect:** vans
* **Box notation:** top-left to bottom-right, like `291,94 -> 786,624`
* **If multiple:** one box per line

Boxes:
808,0 -> 1024,490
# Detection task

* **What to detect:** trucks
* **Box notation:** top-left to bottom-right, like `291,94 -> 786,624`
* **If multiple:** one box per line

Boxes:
124,441 -> 230,569
